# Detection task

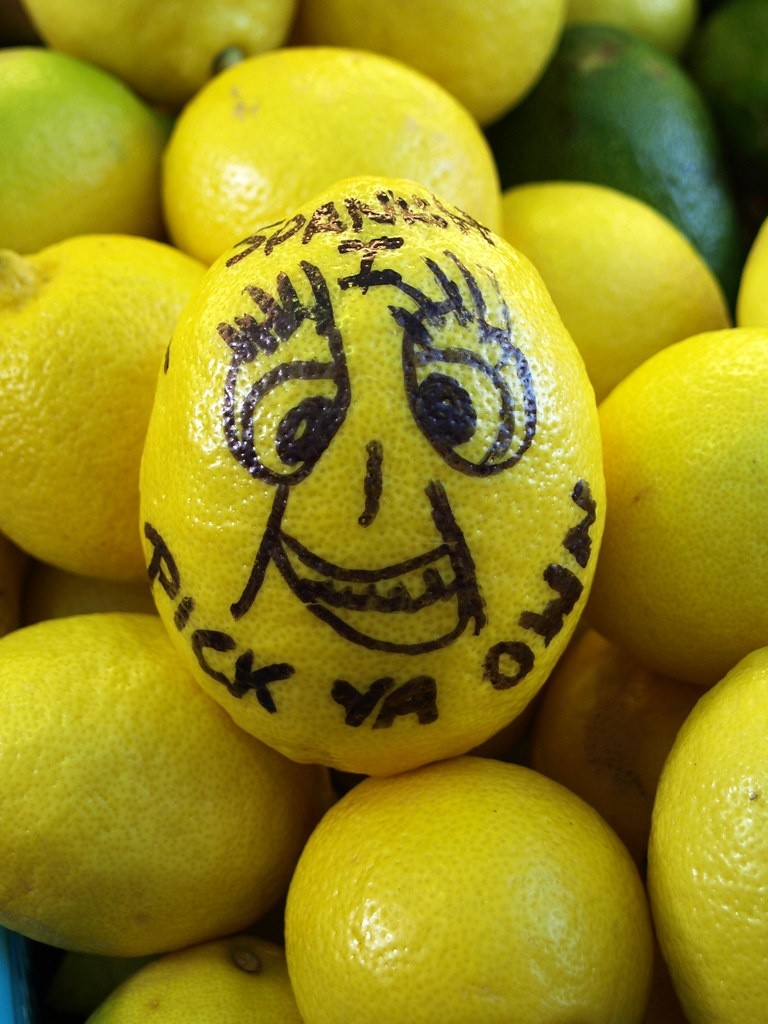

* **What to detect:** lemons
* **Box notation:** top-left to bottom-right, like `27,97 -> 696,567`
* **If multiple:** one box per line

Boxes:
2,0 -> 767,1024
